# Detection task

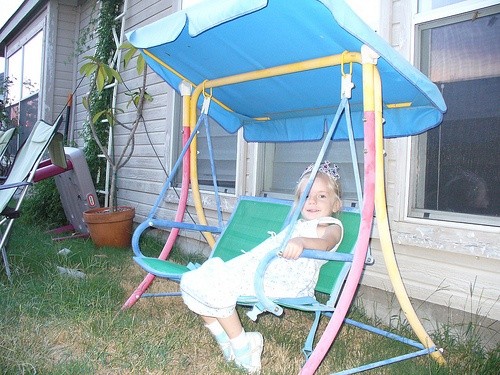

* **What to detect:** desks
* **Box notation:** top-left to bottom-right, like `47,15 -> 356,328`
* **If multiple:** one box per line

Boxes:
29,147 -> 99,241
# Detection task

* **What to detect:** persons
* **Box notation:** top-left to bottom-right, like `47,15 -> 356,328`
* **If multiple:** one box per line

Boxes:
179,161 -> 345,375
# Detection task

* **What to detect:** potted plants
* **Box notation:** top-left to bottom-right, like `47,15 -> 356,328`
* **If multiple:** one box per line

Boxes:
79,42 -> 147,248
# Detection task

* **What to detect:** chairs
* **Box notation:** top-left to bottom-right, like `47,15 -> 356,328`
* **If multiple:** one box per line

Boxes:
0,115 -> 68,284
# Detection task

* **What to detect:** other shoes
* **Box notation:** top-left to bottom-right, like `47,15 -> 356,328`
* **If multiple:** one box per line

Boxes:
234,332 -> 264,375
217,341 -> 233,373
